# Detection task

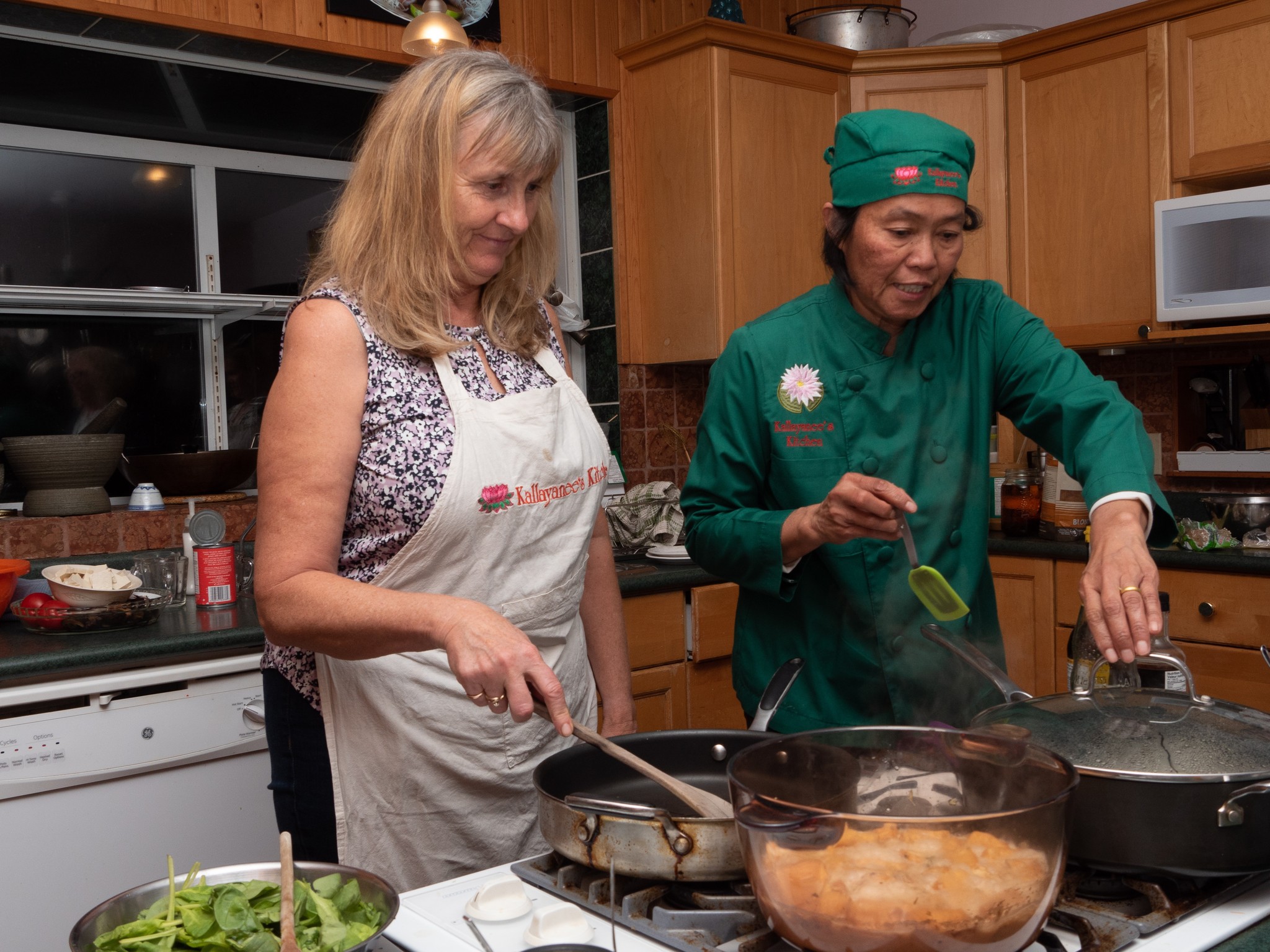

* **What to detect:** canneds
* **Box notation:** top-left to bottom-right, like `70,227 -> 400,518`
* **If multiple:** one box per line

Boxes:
188,510 -> 236,609
1001,468 -> 1044,537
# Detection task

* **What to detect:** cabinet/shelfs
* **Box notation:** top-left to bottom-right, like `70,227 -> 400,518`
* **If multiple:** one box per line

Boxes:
684,581 -> 747,731
850,42 -> 1012,298
1003,2 -> 1179,352
1053,561 -> 1270,715
612,17 -> 860,368
988,554 -> 1055,698
596,591 -> 689,734
1166,0 -> 1270,187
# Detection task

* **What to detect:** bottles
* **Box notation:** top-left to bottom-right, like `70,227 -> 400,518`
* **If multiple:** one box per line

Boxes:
1040,453 -> 1048,475
1000,467 -> 1043,543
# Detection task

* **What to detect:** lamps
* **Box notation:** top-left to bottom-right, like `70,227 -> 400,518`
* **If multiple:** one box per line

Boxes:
401,0 -> 469,58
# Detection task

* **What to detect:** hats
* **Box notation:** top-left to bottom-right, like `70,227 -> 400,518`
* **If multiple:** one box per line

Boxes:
823,109 -> 975,208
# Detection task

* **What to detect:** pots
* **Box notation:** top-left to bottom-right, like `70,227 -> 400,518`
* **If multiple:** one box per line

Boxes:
0,433 -> 123,517
118,432 -> 260,496
540,588 -> 1269,952
785,3 -> 917,51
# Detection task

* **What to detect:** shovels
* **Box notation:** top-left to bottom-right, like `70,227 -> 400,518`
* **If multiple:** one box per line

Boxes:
533,701 -> 733,817
892,483 -> 969,620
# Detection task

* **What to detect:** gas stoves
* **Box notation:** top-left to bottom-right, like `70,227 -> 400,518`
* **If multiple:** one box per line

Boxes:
512,848 -> 1270,951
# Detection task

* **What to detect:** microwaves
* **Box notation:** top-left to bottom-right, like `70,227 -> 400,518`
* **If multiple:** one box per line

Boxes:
1153,182 -> 1270,323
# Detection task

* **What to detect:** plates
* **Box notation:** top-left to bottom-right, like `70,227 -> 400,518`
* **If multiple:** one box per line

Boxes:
10,587 -> 174,635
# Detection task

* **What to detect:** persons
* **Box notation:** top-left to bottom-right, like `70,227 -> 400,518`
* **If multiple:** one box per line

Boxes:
677,105 -> 1181,739
250,48 -> 637,893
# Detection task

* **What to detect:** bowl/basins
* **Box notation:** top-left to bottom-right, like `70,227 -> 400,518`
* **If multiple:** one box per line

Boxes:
0,559 -> 31,618
41,564 -> 142,607
126,483 -> 166,511
68,860 -> 401,952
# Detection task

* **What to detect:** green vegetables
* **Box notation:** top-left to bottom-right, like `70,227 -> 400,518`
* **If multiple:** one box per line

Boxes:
87,854 -> 381,952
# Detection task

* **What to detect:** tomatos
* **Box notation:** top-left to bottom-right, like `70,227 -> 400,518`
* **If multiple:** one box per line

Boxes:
17,592 -> 71,630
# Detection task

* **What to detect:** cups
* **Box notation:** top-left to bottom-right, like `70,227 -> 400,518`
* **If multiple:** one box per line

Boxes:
130,550 -> 189,609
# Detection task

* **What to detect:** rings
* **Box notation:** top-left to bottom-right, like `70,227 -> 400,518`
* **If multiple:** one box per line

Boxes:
485,693 -> 506,707
1120,586 -> 1141,597
466,690 -> 485,700
